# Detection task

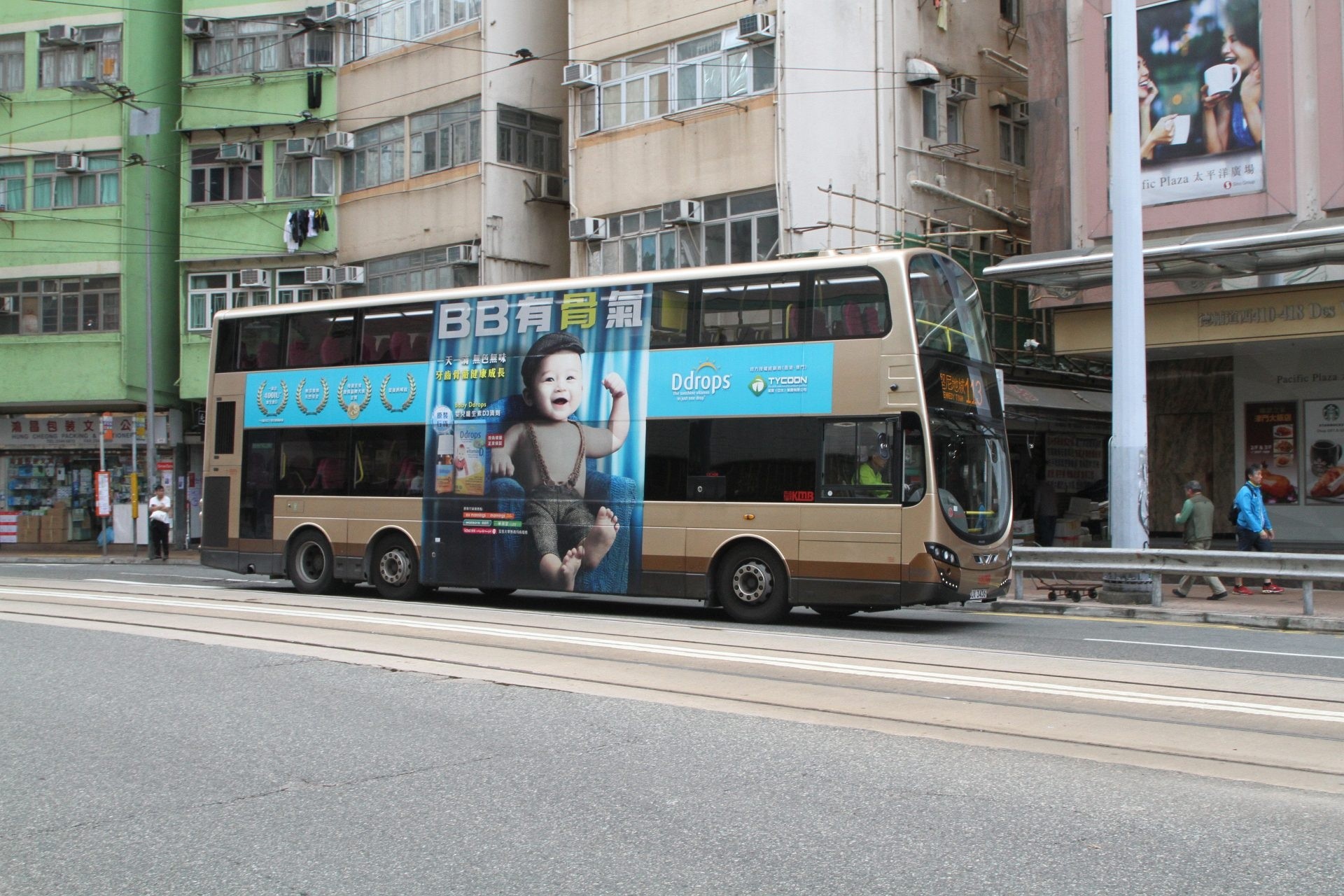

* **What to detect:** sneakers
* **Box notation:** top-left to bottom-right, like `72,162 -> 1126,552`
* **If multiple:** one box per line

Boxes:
1232,585 -> 1254,595
1262,583 -> 1284,594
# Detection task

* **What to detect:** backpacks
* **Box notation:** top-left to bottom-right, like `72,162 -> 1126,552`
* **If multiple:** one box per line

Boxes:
1228,484 -> 1257,525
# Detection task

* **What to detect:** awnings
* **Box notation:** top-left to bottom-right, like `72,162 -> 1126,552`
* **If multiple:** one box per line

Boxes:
983,224 -> 1343,292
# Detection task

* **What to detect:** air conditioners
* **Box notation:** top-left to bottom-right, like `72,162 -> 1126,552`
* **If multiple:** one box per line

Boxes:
737,14 -> 775,40
183,17 -> 213,38
662,200 -> 700,225
446,245 -> 478,267
536,173 -> 570,202
305,2 -> 356,26
220,143 -> 253,162
334,267 -> 365,285
1013,102 -> 1028,124
286,138 -> 317,159
240,269 -> 270,287
55,153 -> 88,173
0,296 -> 11,314
49,24 -> 79,45
568,218 -> 606,241
948,77 -> 978,102
562,62 -> 600,89
304,267 -> 334,284
325,133 -> 356,151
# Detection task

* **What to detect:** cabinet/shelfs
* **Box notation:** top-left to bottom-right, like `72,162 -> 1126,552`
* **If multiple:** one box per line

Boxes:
8,464 -> 70,509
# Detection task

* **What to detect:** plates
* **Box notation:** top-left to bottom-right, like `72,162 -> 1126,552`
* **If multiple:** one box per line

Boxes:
1273,425 -> 1294,437
1274,440 -> 1294,453
1276,455 -> 1291,466
1306,481 -> 1344,498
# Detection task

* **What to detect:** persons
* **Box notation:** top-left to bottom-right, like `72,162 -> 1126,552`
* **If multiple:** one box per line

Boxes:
852,440 -> 893,499
1230,461 -> 1282,598
1035,473 -> 1057,549
235,320 -> 426,370
1137,0 -> 1264,166
147,484 -> 172,561
974,490 -> 988,536
249,456 -> 425,539
491,332 -> 629,598
1171,481 -> 1227,601
454,444 -> 468,476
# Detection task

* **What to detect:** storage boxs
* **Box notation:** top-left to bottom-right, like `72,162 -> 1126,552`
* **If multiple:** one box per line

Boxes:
8,460 -> 69,544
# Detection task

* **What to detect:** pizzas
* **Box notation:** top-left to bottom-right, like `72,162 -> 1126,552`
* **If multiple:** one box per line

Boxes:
1310,465 -> 1344,498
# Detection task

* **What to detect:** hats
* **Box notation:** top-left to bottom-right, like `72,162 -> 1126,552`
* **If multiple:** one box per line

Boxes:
521,333 -> 585,387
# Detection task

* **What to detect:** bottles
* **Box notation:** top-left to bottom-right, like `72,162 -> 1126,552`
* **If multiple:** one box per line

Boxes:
435,434 -> 454,493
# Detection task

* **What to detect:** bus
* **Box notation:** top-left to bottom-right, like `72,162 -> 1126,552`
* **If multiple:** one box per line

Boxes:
199,248 -> 1013,626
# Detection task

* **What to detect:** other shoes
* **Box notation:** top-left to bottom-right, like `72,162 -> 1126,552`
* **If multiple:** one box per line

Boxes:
1174,589 -> 1186,597
162,555 -> 169,560
1207,591 -> 1227,600
150,555 -> 161,559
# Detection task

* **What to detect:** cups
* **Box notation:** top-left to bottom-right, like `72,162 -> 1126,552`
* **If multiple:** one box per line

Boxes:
1168,114 -> 1190,145
1310,439 -> 1343,476
1204,64 -> 1241,97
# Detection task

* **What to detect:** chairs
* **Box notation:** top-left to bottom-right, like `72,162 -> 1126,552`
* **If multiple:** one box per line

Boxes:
651,300 -> 883,348
249,450 -> 425,496
239,327 -> 432,371
